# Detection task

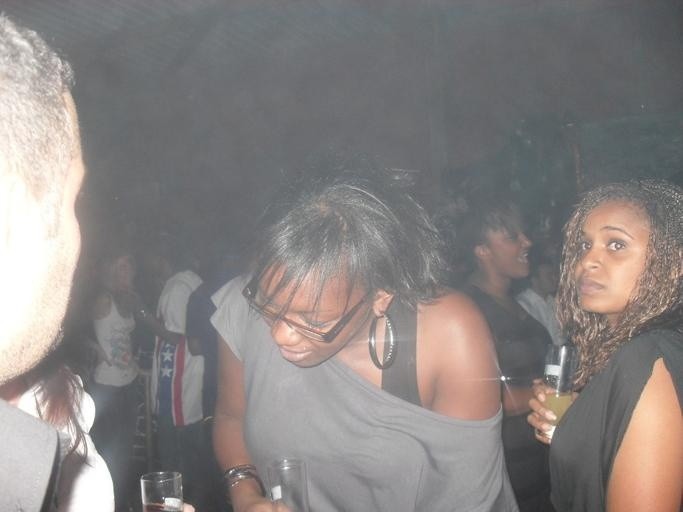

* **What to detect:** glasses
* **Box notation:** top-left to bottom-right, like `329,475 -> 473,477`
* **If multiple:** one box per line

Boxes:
240,262 -> 372,343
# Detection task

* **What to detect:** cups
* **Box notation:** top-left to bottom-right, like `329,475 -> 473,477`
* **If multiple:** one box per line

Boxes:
534,345 -> 583,447
140,470 -> 184,512
266,458 -> 311,512
